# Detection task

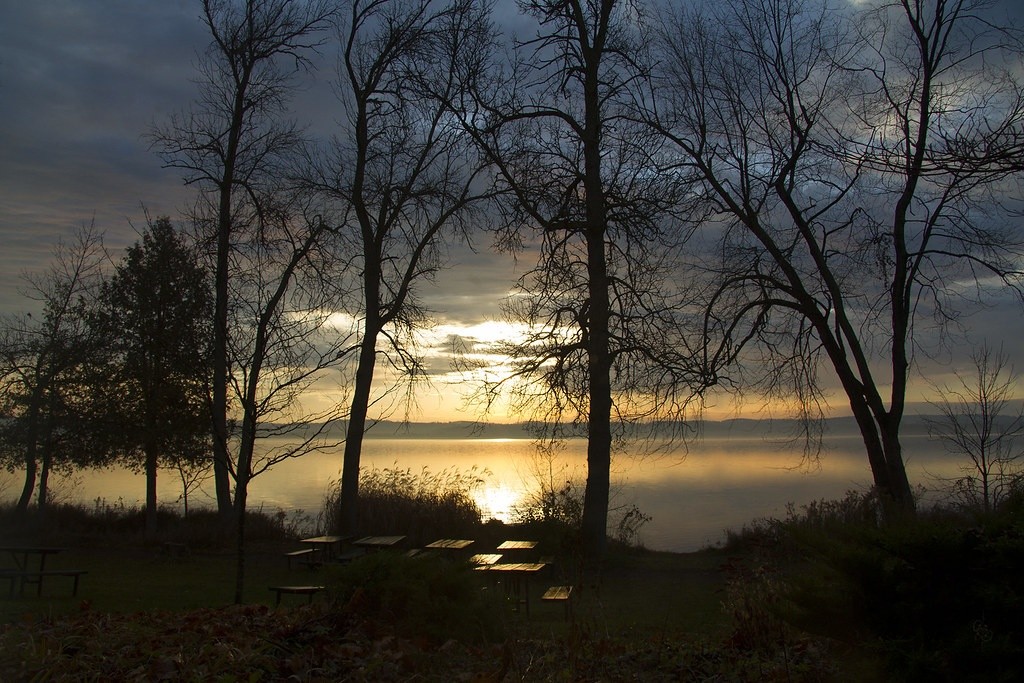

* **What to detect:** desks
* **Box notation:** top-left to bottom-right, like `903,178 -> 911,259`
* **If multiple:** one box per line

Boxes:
497,540 -> 539,594
351,536 -> 408,551
0,546 -> 70,596
472,564 -> 545,618
425,539 -> 475,550
300,536 -> 350,560
268,586 -> 328,606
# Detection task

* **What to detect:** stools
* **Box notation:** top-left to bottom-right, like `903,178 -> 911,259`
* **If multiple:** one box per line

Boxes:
539,556 -> 560,578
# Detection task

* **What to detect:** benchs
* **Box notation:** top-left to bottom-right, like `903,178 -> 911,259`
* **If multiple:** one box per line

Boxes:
541,586 -> 574,623
0,568 -> 88,601
400,549 -> 421,558
284,549 -> 320,570
466,552 -> 503,565
